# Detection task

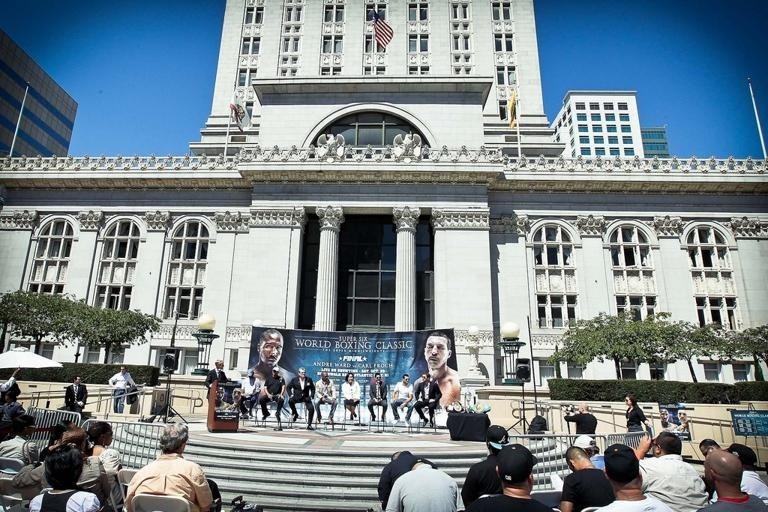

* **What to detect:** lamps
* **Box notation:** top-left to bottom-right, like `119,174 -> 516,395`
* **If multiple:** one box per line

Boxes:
495,319 -> 528,386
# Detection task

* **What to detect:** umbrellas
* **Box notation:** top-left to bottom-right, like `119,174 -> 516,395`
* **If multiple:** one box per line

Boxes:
0,346 -> 64,369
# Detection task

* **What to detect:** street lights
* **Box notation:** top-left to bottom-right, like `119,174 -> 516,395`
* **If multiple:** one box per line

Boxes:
464,324 -> 487,378
497,322 -> 531,387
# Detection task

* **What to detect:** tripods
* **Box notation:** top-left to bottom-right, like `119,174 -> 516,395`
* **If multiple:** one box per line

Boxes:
151,374 -> 188,424
506,382 -> 530,435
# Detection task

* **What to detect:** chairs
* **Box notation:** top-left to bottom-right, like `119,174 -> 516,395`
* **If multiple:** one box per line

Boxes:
475,489 -> 600,512
1,451 -> 193,511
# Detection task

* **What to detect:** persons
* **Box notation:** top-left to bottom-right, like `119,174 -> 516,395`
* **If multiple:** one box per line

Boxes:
239,369 -> 260,417
215,386 -> 241,411
0,365 -> 213,512
367,373 -> 388,423
314,370 -> 338,423
414,373 -> 442,428
248,329 -> 297,397
286,367 -> 315,430
259,367 -> 286,421
341,372 -> 361,421
390,373 -> 414,427
204,359 -> 229,399
414,331 -> 461,408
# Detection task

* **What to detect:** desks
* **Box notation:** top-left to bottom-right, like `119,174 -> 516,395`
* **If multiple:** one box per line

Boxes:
445,411 -> 489,441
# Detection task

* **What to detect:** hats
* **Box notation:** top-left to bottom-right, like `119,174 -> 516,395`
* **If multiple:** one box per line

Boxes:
728,444 -> 757,465
604,444 -> 639,482
487,425 -> 537,482
573,435 -> 595,449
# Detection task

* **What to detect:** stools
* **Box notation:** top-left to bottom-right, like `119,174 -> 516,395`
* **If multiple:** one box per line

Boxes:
248,399 -> 442,433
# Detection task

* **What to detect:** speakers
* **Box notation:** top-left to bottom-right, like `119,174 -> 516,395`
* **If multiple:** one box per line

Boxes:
516,358 -> 530,382
163,348 -> 180,374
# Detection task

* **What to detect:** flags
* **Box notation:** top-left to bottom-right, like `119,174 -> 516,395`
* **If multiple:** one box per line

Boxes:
229,89 -> 252,133
508,88 -> 517,129
372,10 -> 394,50
